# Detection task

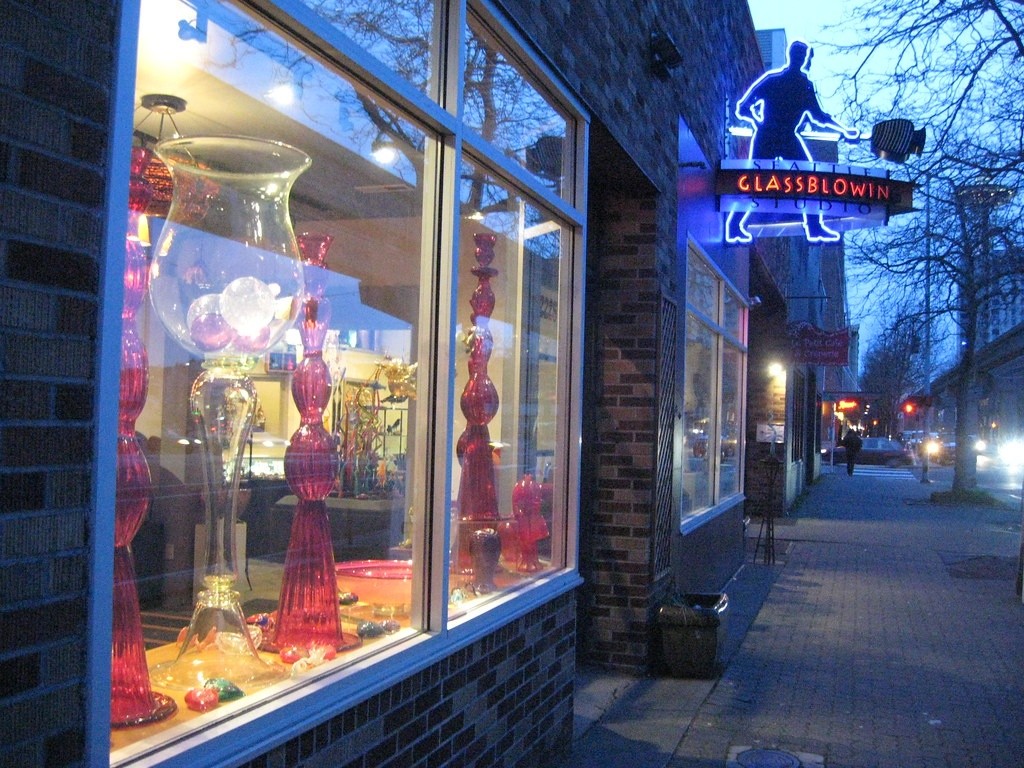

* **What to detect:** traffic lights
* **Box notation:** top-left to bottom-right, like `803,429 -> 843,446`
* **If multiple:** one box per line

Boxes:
909,335 -> 922,354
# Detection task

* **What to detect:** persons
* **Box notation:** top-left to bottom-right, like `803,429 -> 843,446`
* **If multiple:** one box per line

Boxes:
840,429 -> 863,476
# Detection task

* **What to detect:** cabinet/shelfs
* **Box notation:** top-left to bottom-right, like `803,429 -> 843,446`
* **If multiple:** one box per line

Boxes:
268,380 -> 410,567
221,433 -> 294,560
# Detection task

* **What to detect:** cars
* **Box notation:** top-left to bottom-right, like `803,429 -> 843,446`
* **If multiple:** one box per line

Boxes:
820,437 -> 911,470
899,430 -> 986,466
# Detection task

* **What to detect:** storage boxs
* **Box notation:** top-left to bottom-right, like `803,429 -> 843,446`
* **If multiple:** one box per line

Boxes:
757,420 -> 785,444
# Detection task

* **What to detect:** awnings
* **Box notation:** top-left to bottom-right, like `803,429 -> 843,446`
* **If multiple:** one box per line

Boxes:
827,389 -> 880,405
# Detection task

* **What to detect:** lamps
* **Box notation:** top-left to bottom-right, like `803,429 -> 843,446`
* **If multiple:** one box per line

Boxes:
648,15 -> 683,82
339,117 -> 354,132
177,19 -> 196,41
